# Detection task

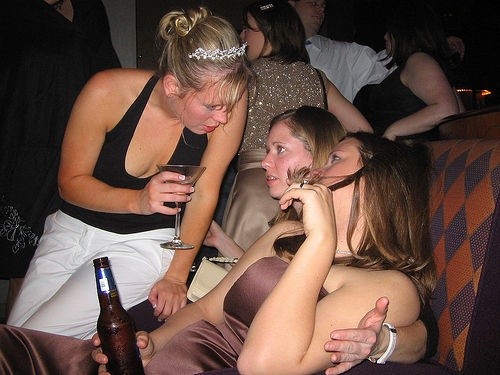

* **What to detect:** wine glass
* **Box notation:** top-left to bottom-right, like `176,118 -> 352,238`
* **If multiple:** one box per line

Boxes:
155,165 -> 206,249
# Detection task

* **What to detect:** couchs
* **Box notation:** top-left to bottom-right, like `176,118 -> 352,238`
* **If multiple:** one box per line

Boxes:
196,105 -> 500,375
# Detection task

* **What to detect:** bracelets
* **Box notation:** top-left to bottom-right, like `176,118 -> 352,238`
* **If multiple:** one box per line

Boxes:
365,324 -> 397,366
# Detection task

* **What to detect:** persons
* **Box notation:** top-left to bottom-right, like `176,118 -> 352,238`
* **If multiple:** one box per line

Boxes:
1,0 -> 491,375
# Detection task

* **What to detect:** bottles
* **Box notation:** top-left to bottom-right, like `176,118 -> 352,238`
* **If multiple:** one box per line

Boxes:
93,256 -> 146,375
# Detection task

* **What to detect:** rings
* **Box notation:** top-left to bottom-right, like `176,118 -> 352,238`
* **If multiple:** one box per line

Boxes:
299,182 -> 305,188
